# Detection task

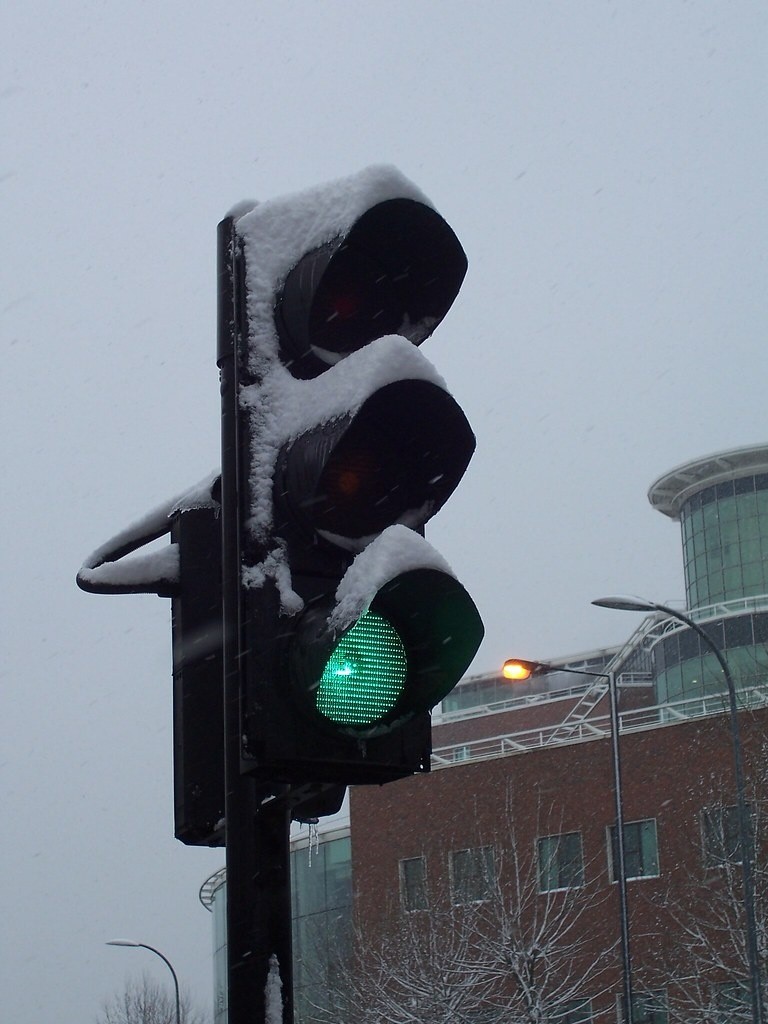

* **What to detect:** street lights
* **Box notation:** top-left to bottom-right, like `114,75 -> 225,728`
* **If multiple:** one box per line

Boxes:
109,941 -> 182,1024
592,595 -> 768,1023
500,660 -> 636,1024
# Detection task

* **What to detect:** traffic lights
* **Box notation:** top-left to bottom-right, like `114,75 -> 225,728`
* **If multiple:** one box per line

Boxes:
222,188 -> 488,818
